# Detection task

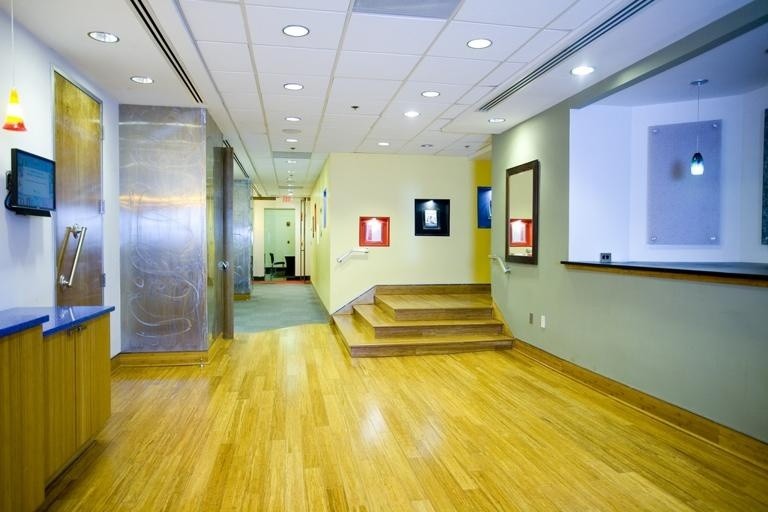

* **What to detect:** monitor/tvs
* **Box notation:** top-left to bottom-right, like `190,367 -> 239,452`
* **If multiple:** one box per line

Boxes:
11,149 -> 56,212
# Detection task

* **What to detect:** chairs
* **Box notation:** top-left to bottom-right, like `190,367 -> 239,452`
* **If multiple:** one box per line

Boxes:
269,253 -> 285,275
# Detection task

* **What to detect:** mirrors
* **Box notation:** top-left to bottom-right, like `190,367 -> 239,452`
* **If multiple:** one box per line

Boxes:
506,159 -> 539,265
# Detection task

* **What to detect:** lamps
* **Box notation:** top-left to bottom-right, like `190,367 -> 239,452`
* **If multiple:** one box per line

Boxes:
690,79 -> 709,176
2,1 -> 27,131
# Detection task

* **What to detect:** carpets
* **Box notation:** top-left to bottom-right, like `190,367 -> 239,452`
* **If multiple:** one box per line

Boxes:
251,280 -> 310,284
273,272 -> 285,278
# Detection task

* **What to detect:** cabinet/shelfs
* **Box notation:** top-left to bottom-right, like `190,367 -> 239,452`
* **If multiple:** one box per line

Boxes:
1,306 -> 115,511
